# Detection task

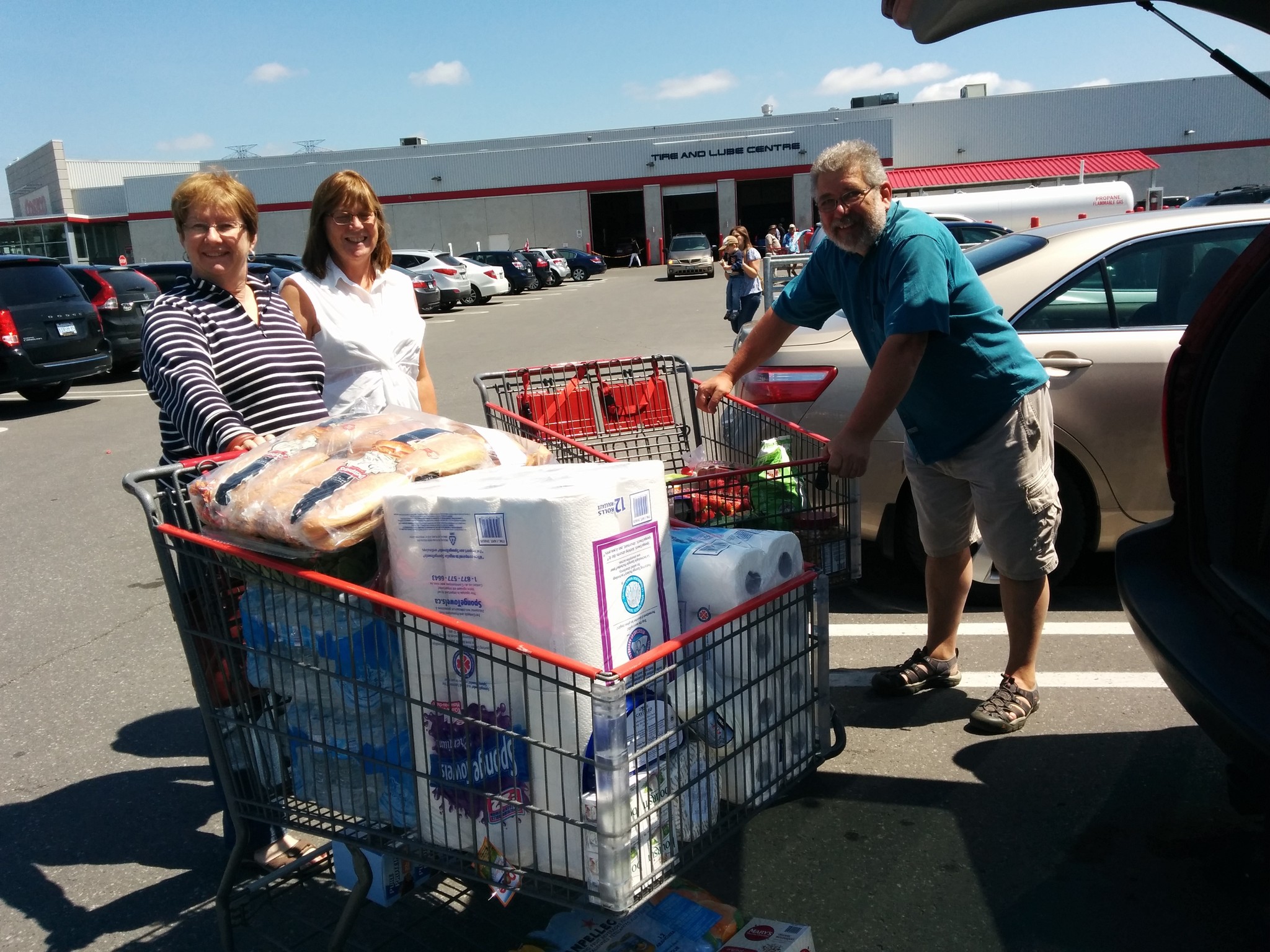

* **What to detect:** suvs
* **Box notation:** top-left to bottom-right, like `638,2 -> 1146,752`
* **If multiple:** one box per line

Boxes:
122,253 -> 307,294
0,253 -> 114,403
60,264 -> 162,383
457,250 -> 536,296
514,247 -> 571,288
520,252 -> 553,291
390,249 -> 471,313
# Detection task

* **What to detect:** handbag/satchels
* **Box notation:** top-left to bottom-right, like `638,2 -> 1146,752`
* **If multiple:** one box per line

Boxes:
760,279 -> 764,295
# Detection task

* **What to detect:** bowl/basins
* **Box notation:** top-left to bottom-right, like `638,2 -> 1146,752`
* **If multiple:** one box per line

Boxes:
680,460 -> 752,523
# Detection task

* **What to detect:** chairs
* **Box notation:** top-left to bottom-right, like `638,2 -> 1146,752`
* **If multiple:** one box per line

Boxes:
1177,246 -> 1239,325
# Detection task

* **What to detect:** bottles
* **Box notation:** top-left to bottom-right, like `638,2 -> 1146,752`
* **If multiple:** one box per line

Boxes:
286,701 -> 338,808
338,593 -> 403,713
240,583 -> 293,696
793,512 -> 848,584
328,713 -> 417,828
287,587 -> 338,707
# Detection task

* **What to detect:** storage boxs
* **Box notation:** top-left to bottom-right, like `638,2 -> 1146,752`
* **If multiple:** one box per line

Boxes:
716,916 -> 815,952
199,523 -> 379,595
331,821 -> 453,908
578,761 -> 680,908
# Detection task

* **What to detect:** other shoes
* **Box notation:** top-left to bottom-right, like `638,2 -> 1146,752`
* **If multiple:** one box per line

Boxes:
791,271 -> 797,275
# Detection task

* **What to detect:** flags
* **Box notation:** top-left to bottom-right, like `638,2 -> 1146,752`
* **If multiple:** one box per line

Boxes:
524,238 -> 530,252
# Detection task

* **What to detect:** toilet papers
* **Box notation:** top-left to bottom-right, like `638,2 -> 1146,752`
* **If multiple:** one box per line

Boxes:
380,458 -> 813,885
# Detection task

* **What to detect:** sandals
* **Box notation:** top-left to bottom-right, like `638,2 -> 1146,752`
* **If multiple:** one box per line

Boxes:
724,309 -> 731,320
870,645 -> 962,696
970,673 -> 1041,734
728,310 -> 739,321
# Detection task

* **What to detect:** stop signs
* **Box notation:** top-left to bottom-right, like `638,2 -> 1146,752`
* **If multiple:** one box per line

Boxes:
119,255 -> 127,267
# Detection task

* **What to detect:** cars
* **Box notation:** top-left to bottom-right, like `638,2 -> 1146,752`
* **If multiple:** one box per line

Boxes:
662,232 -> 718,281
453,257 -> 510,307
557,248 -> 608,282
390,264 -> 441,314
719,180 -> 1269,784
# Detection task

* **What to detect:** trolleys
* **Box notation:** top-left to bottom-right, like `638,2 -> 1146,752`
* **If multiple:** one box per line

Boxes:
471,351 -> 864,587
119,446 -> 847,951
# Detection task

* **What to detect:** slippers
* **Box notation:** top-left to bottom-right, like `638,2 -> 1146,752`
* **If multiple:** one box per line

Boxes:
252,835 -> 333,877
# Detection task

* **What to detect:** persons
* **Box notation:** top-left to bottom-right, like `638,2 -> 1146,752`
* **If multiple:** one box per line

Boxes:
628,238 -> 642,268
277,170 -> 439,416
695,139 -> 1062,733
140,170 -> 330,872
718,224 -> 811,334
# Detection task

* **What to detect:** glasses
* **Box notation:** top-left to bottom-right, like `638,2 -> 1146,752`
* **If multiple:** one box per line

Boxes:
734,234 -> 742,238
326,211 -> 378,225
818,183 -> 876,213
182,220 -> 249,236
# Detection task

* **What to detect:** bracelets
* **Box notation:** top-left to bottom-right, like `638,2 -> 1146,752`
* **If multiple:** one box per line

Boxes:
742,263 -> 746,268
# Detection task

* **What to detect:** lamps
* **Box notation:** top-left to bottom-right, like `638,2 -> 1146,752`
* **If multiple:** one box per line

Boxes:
430,176 -> 442,182
1184,130 -> 1195,135
957,149 -> 966,154
646,162 -> 655,166
798,149 -> 807,155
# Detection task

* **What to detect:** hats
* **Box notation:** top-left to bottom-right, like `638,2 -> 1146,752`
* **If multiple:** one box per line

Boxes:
789,224 -> 795,229
718,235 -> 738,251
769,225 -> 777,230
